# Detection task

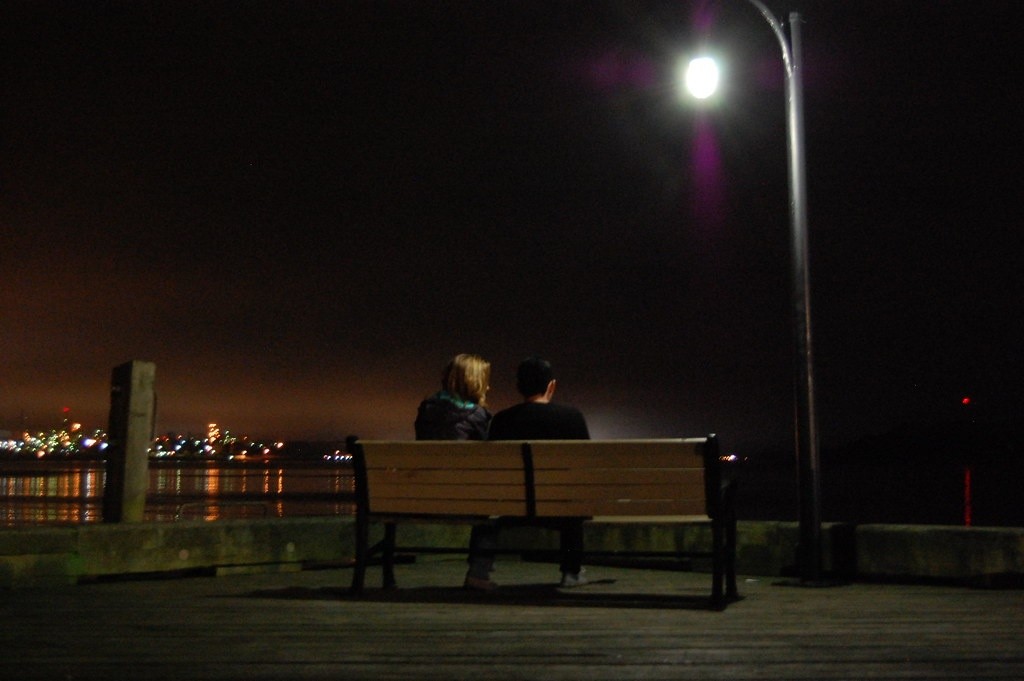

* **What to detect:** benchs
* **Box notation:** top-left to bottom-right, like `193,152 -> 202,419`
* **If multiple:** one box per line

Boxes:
344,435 -> 740,610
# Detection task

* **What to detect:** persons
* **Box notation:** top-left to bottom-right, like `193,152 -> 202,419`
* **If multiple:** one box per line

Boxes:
411,353 -> 512,598
484,355 -> 595,589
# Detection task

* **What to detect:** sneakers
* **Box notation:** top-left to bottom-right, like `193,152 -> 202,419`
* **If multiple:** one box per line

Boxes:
461,567 -> 506,597
554,570 -> 605,595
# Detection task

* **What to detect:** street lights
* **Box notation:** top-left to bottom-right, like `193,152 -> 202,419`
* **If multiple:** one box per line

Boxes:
688,0 -> 835,585
